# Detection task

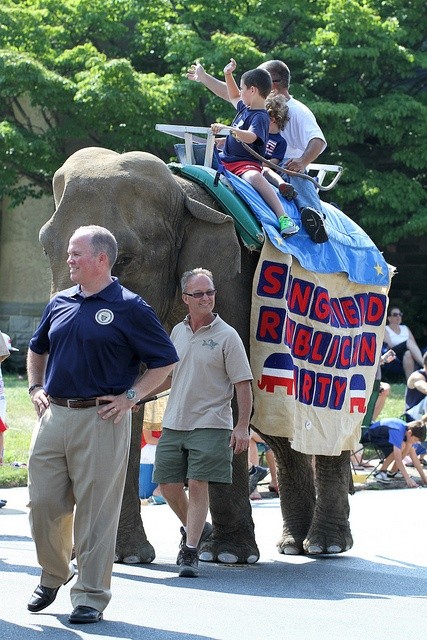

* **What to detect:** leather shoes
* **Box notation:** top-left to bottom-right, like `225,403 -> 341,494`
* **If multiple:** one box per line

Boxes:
26,572 -> 75,612
69,605 -> 103,624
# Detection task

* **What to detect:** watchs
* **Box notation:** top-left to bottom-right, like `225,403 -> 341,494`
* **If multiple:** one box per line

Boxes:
125,389 -> 140,404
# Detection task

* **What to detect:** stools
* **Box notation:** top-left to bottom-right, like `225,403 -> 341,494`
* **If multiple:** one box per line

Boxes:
351,441 -> 385,481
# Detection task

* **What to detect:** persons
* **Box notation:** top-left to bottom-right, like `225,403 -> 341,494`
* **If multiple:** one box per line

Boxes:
210,59 -> 299,235
405,416 -> 426,467
382,307 -> 424,382
262,92 -> 299,200
369,348 -> 396,426
248,428 -> 277,500
186,60 -> 330,242
0,332 -> 9,508
358,418 -> 426,488
26,226 -> 179,624
131,268 -> 253,577
404,351 -> 426,423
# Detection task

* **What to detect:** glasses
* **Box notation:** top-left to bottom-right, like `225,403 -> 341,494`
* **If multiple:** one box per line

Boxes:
183,290 -> 215,298
390,313 -> 403,317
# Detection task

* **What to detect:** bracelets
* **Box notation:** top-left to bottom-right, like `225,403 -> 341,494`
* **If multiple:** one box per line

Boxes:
29,384 -> 43,393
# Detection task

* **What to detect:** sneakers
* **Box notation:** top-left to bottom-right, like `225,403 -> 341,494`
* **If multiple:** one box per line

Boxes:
372,470 -> 391,485
278,183 -> 298,201
300,207 -> 328,243
177,545 -> 200,577
176,522 -> 213,565
386,471 -> 403,478
248,465 -> 270,495
278,213 -> 300,240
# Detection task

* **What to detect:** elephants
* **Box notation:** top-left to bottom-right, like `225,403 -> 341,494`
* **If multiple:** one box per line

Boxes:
38,143 -> 357,566
